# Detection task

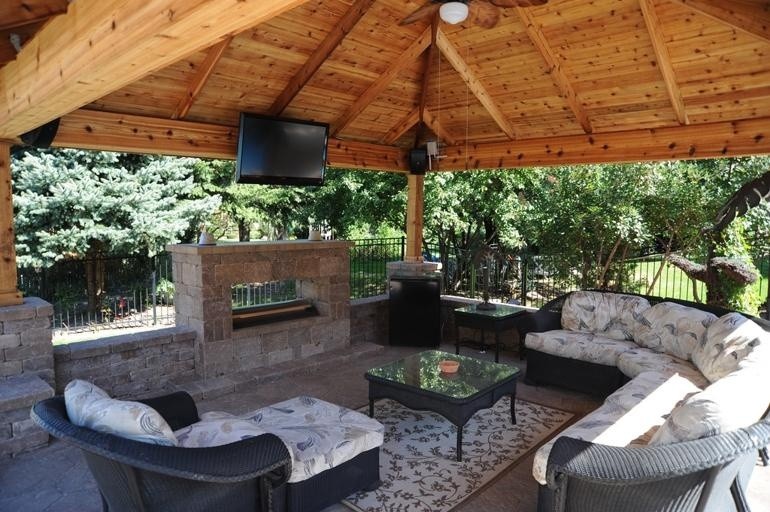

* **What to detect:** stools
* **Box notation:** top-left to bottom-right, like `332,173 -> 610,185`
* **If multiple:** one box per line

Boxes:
236,392 -> 385,510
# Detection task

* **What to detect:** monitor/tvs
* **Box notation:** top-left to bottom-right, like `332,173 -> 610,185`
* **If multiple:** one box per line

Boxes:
236,112 -> 330,186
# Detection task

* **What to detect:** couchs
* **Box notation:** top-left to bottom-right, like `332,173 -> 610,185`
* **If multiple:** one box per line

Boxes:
515,286 -> 769,511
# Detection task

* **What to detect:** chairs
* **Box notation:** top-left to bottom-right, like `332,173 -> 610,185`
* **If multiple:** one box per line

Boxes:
28,379 -> 293,511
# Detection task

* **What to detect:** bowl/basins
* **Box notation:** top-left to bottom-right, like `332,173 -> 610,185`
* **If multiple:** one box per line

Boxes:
439,360 -> 459,374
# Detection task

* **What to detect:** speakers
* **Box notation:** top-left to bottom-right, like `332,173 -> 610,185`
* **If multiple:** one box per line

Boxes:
409,149 -> 426,175
388,275 -> 440,348
21,117 -> 60,150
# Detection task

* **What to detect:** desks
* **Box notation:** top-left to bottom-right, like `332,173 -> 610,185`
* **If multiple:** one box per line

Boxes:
452,302 -> 526,363
